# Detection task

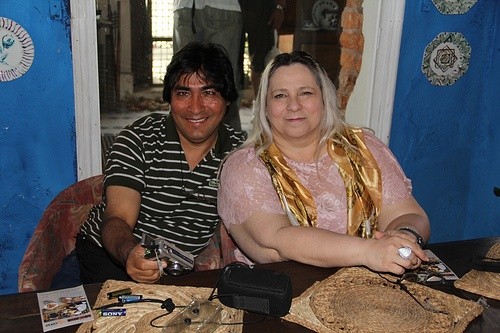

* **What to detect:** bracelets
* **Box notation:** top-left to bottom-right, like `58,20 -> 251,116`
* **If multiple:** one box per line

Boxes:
276,5 -> 284,10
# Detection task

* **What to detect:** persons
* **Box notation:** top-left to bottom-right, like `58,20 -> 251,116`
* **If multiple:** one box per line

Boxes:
195,49 -> 430,275
172,0 -> 287,102
47,41 -> 249,290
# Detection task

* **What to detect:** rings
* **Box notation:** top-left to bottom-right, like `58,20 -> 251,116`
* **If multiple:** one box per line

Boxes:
398,247 -> 411,259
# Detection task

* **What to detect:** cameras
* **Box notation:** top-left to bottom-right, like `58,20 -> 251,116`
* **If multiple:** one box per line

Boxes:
144,237 -> 194,276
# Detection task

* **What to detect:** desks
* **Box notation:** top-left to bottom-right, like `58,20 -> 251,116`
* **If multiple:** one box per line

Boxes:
0,237 -> 500,333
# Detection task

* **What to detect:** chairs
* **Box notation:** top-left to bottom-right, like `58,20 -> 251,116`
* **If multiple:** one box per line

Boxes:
18,177 -> 221,292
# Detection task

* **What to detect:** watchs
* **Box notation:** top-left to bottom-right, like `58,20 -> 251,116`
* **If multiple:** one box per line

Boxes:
398,227 -> 424,249
